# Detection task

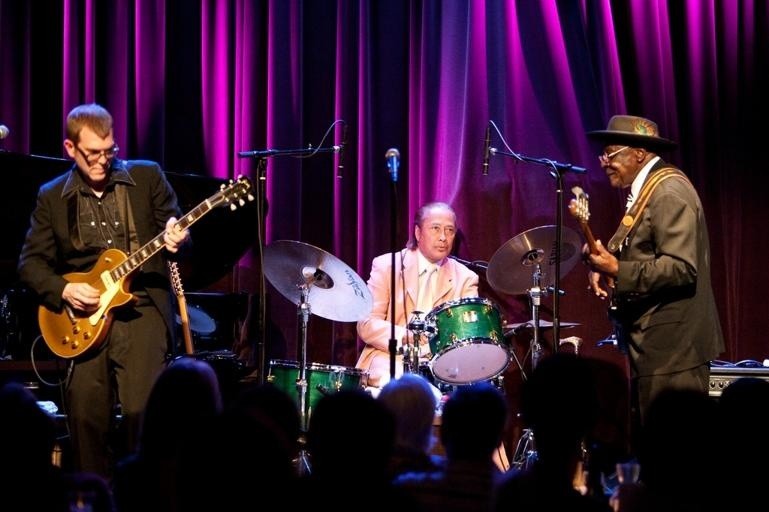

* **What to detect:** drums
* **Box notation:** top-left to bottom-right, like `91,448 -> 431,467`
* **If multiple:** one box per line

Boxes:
403,357 -> 435,389
422,297 -> 512,385
268,358 -> 367,411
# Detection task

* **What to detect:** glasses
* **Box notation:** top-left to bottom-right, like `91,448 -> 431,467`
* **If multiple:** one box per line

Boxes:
75,142 -> 119,167
599,146 -> 630,167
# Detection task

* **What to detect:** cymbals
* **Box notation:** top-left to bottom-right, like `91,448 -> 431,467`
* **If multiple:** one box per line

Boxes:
503,317 -> 582,331
487,227 -> 583,294
261,241 -> 373,322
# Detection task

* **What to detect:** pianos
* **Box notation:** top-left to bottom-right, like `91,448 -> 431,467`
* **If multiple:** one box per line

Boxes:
1,148 -> 268,360
708,368 -> 769,396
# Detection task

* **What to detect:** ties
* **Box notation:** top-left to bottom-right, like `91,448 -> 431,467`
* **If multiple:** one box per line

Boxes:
417,264 -> 436,321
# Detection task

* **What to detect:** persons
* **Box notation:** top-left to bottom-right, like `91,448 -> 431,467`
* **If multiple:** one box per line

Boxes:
17,103 -> 191,474
353,201 -> 482,396
583,115 -> 726,424
3,357 -> 768,511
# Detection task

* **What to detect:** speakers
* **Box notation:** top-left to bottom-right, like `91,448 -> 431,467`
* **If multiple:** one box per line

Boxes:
167,287 -> 249,357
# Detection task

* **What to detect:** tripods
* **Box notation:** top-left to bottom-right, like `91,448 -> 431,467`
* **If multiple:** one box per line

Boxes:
508,282 -> 545,471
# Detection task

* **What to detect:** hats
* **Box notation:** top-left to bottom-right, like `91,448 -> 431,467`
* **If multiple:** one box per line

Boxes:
586,115 -> 675,157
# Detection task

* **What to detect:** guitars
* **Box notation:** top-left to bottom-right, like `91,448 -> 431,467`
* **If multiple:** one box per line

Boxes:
569,185 -> 628,352
38,172 -> 256,360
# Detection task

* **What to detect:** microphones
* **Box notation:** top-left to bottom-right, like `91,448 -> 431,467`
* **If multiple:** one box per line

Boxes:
482,128 -> 490,176
337,126 -> 348,179
0,126 -> 10,140
385,148 -> 401,184
